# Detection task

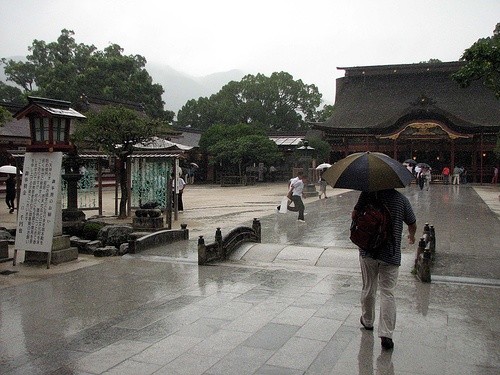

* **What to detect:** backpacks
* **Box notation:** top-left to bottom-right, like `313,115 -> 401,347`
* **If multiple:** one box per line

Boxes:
349,191 -> 396,257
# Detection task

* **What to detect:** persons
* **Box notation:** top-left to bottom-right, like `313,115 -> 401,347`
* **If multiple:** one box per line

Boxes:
318,167 -> 329,199
189,165 -> 195,184
402,162 -> 431,191
277,172 -> 309,223
452,165 -> 467,185
441,165 -> 450,185
171,173 -> 186,210
350,188 -> 417,349
491,166 -> 498,183
3,173 -> 16,214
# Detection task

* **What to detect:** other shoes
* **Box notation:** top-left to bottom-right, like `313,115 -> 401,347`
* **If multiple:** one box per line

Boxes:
360,315 -> 374,331
179,211 -> 183,212
277,206 -> 281,210
298,219 -> 305,223
9,207 -> 13,213
381,337 -> 394,349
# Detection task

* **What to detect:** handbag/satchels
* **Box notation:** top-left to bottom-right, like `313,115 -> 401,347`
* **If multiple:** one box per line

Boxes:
279,197 -> 292,214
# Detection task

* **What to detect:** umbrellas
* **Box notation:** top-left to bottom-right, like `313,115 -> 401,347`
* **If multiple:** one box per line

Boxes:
190,162 -> 199,168
405,159 -> 416,164
322,150 -> 413,191
0,164 -> 23,174
316,163 -> 332,170
417,163 -> 430,169
